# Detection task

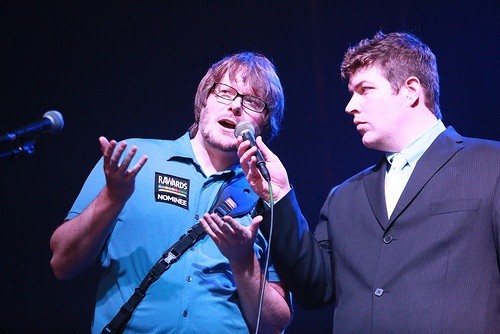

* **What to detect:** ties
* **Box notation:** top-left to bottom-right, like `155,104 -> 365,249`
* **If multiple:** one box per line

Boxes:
385,155 -> 409,217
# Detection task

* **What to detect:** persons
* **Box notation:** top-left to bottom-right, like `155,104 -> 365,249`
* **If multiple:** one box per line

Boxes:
235,32 -> 500,334
48,50 -> 306,334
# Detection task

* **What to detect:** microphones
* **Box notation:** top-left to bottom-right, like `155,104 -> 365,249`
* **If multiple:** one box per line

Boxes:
234,121 -> 271,181
0,111 -> 64,143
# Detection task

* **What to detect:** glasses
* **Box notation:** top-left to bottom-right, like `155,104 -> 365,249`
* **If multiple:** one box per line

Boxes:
211,81 -> 269,114
